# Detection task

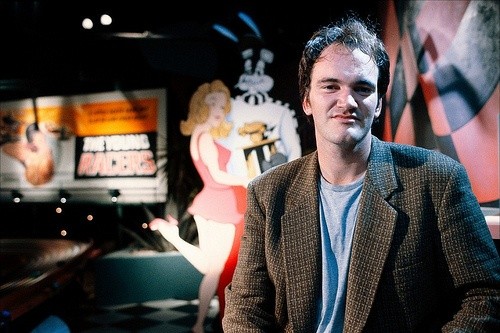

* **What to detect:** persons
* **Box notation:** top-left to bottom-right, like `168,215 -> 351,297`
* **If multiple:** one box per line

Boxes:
150,80 -> 253,333
226,20 -> 500,333
